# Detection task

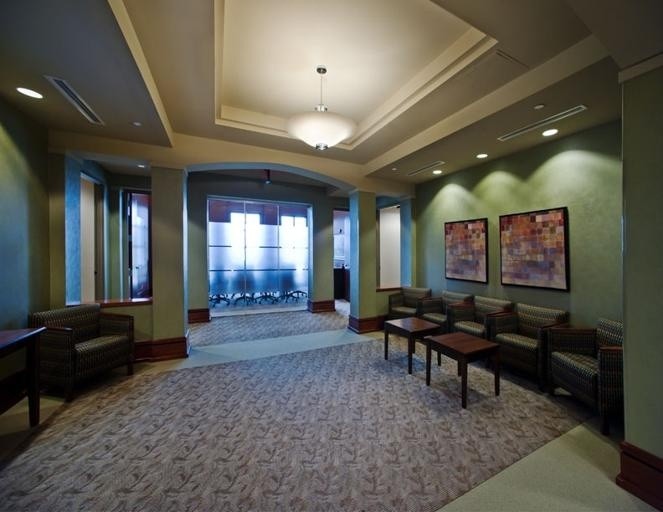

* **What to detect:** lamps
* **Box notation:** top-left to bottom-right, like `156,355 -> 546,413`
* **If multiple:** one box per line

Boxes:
284,66 -> 359,151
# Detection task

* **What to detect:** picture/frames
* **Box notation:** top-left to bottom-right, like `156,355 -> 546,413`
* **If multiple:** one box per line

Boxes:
444,206 -> 571,292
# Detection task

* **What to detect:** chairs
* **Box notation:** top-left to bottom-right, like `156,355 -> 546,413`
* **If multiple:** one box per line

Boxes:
388,286 -> 622,436
27,302 -> 134,401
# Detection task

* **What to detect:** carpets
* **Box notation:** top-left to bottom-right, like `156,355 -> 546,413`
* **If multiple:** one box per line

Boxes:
0,334 -> 597,512
186,298 -> 350,346
